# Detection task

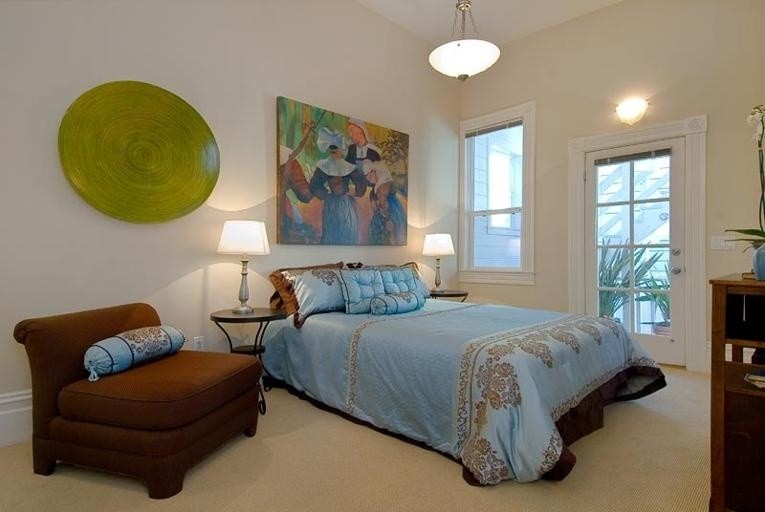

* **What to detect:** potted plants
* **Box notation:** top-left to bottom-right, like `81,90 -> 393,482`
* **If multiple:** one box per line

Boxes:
635,269 -> 670,337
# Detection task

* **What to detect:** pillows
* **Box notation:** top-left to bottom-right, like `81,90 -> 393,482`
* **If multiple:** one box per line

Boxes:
268,261 -> 432,331
82,321 -> 188,384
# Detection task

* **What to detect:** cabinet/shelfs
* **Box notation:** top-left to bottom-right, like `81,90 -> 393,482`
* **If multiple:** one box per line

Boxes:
706,270 -> 765,511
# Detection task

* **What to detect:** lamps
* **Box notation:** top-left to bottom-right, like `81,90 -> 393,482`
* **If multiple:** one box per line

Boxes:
422,231 -> 455,293
215,218 -> 273,314
426,1 -> 501,85
613,96 -> 650,127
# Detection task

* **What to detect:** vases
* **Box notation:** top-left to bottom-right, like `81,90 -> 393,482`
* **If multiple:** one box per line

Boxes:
751,243 -> 765,282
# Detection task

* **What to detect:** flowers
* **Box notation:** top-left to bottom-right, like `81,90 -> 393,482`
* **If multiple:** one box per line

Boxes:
723,101 -> 764,253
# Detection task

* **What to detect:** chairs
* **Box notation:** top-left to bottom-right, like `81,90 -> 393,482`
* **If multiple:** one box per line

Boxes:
10,302 -> 267,499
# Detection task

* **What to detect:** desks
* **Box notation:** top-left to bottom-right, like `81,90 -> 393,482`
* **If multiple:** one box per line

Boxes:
432,289 -> 470,304
208,304 -> 281,415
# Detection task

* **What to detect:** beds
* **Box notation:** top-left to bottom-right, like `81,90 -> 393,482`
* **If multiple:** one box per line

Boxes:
258,260 -> 669,482
